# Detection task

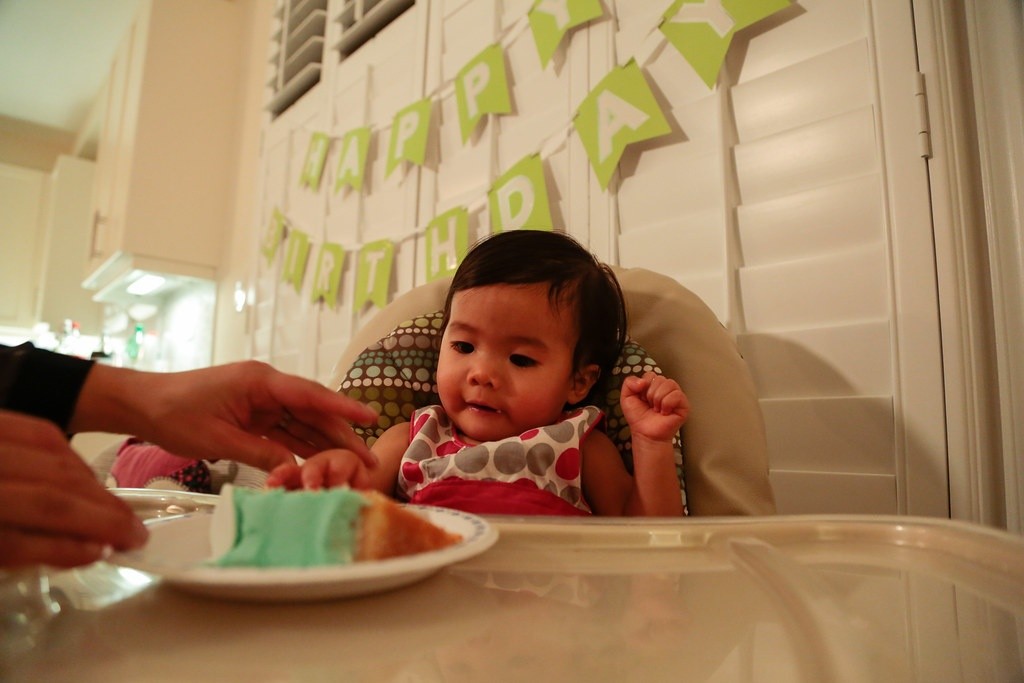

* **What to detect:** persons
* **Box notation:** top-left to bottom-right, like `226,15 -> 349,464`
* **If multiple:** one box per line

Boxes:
0,337 -> 382,581
264,228 -> 692,518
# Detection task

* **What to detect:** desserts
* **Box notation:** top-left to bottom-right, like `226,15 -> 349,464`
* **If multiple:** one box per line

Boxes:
202,483 -> 464,567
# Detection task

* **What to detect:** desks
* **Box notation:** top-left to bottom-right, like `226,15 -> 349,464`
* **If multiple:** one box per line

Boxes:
0,487 -> 1024,683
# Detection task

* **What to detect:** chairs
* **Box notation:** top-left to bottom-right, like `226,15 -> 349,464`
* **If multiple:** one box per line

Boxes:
304,263 -> 777,521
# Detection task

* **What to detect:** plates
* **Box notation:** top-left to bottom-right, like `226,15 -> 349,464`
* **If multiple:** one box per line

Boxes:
107,505 -> 499,599
